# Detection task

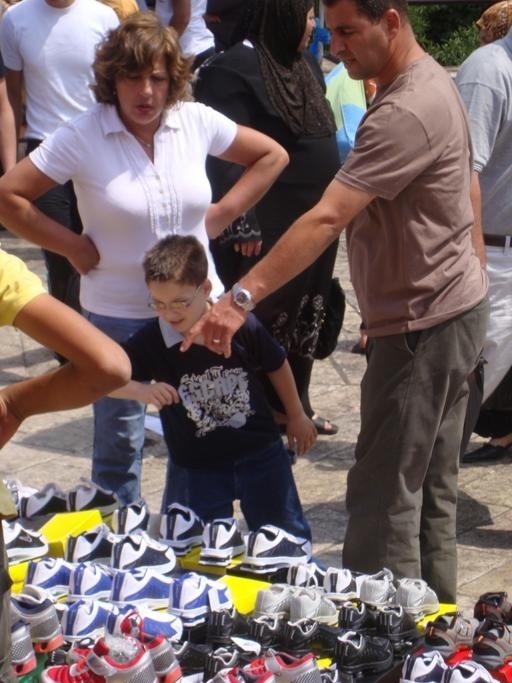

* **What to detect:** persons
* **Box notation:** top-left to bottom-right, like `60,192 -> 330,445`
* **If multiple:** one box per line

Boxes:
105,231 -> 317,545
0,1 -> 510,464
1,244 -> 133,682
0,9 -> 290,512
178,0 -> 490,608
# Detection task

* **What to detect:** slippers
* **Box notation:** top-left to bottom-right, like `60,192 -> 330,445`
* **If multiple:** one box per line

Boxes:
274,416 -> 338,435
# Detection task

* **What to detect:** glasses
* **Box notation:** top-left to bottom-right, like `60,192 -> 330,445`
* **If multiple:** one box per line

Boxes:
147,284 -> 202,311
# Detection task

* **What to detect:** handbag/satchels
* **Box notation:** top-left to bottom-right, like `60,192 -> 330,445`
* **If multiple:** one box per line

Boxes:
314,278 -> 345,359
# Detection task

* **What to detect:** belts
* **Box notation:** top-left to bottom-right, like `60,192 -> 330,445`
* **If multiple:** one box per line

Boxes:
484,232 -> 511,247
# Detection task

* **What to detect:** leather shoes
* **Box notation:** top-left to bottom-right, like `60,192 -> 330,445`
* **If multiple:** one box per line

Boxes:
463,442 -> 511,463
